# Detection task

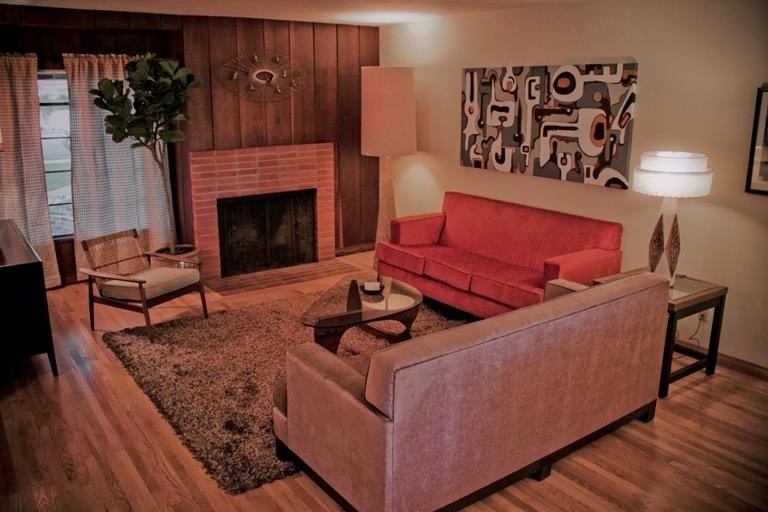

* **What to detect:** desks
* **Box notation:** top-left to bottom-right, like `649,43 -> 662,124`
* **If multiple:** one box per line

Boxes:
592,266 -> 729,398
0,218 -> 59,378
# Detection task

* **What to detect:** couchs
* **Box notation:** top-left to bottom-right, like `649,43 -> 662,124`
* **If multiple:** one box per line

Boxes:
375,191 -> 623,324
273,271 -> 671,512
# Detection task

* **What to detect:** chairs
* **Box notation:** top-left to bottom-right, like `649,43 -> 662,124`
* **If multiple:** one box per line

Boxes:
79,229 -> 209,345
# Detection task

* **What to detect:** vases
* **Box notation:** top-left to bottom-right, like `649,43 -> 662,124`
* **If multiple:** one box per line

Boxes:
89,51 -> 204,294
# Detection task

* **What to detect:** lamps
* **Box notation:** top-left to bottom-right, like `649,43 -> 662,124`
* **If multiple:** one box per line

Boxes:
634,151 -> 712,292
360,66 -> 417,269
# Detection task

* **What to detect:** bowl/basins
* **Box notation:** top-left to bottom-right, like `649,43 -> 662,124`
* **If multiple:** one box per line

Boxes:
360,285 -> 384,295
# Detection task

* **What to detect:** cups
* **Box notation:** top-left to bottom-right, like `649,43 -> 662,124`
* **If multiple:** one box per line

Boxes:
365,282 -> 380,290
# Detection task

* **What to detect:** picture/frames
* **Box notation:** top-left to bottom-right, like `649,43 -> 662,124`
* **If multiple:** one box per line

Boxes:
745,82 -> 768,198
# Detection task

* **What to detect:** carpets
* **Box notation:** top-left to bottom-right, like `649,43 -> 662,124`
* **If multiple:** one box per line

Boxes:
103,290 -> 465,496
